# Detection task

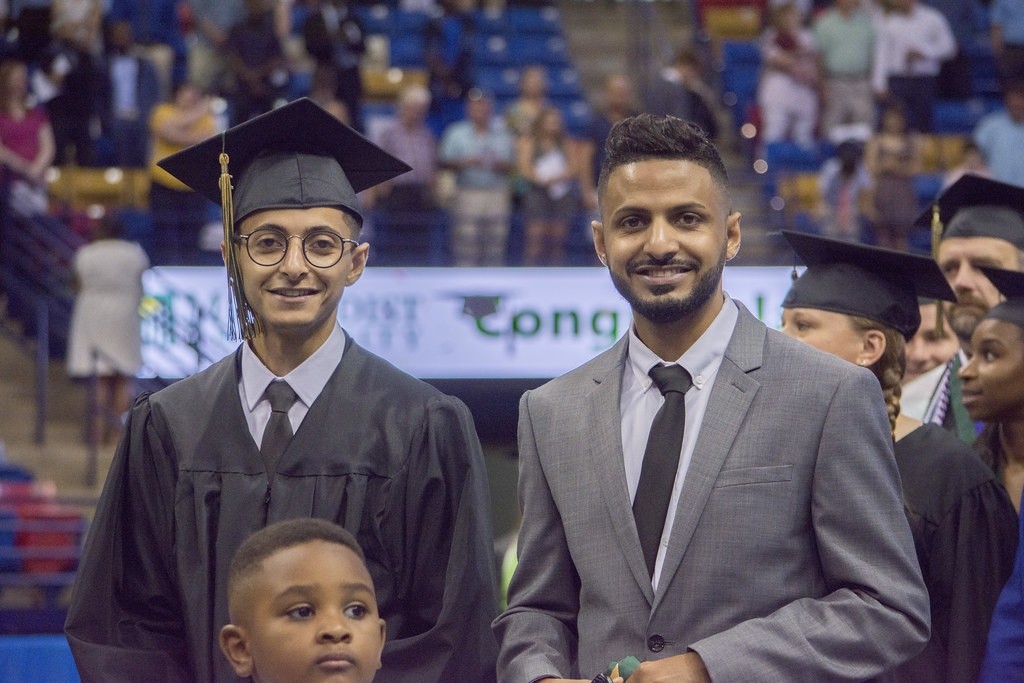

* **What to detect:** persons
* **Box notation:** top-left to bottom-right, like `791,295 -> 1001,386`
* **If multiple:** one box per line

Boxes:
218,516 -> 387,683
956,266 -> 1024,511
62,96 -> 500,683
896,173 -> 1024,447
751,0 -> 1024,244
0,0 -> 365,218
490,114 -> 932,683
372,49 -> 723,270
778,229 -> 1020,683
61,215 -> 149,444
899,297 -> 961,382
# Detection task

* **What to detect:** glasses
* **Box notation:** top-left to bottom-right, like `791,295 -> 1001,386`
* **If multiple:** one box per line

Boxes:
232,228 -> 359,269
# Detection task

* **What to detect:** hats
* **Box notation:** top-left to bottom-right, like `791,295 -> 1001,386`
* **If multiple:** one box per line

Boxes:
975,265 -> 1024,327
781,229 -> 958,342
155,96 -> 415,340
912,172 -> 1024,261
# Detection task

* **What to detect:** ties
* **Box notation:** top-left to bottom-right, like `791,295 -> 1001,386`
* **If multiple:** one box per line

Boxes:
632,364 -> 693,582
260,380 -> 300,486
